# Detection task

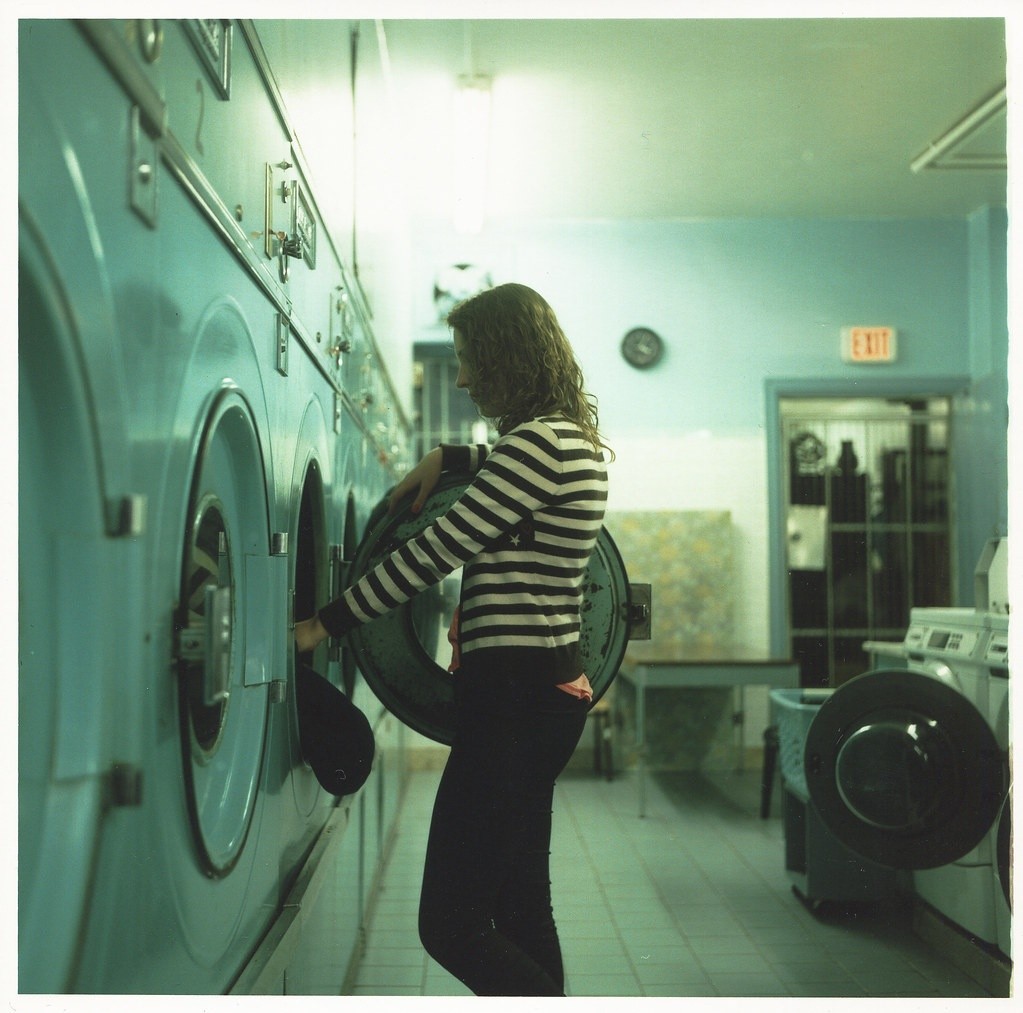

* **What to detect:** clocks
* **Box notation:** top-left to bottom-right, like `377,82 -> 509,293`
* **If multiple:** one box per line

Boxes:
621,327 -> 663,368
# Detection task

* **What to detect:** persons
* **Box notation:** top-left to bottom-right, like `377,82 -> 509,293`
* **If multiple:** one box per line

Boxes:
287,283 -> 615,998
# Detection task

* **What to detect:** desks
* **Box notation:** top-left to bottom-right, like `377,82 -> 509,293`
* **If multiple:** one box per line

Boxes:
606,643 -> 800,821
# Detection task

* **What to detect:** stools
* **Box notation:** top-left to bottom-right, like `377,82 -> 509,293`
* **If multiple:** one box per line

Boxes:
586,700 -> 615,781
